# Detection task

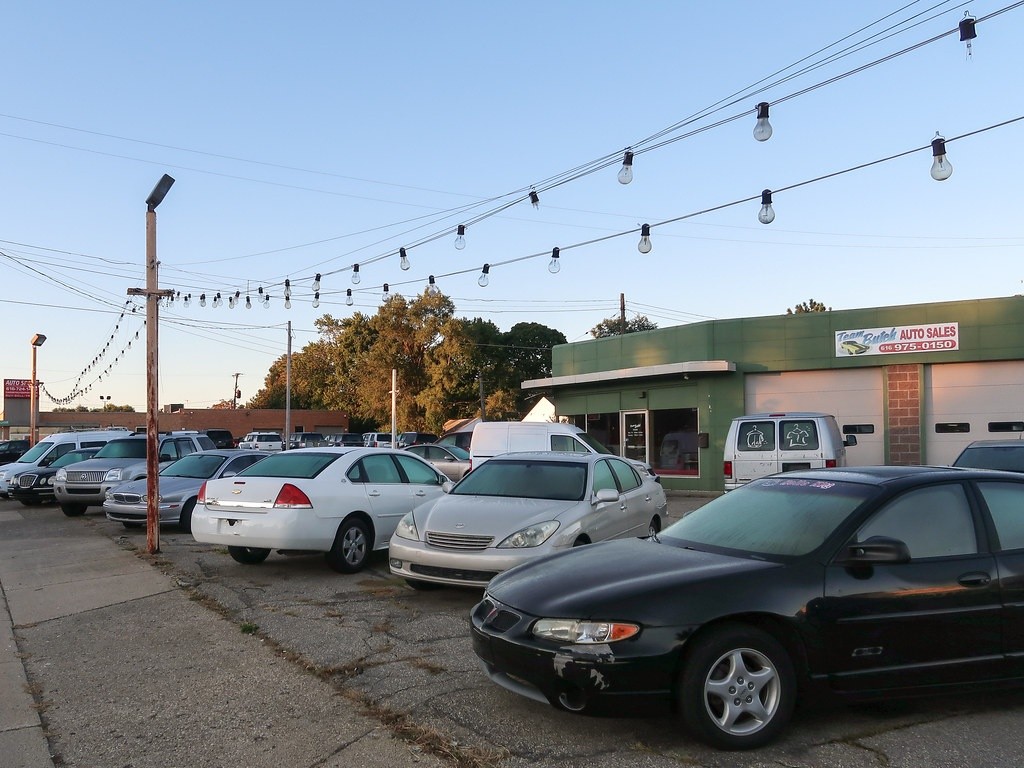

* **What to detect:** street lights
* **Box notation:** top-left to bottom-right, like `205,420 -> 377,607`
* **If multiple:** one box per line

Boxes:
30,334 -> 47,447
100,396 -> 111,412
145,173 -> 176,553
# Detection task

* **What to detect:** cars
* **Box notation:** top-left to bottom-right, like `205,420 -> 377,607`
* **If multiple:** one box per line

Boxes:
199,428 -> 235,449
0,440 -> 30,465
470,466 -> 1024,750
284,432 -> 328,450
660,432 -> 699,471
400,443 -> 470,482
7,447 -> 103,505
432,431 -> 473,453
398,432 -> 438,448
324,433 -> 364,446
103,449 -> 275,533
951,439 -> 1024,473
362,433 -> 399,448
237,431 -> 282,451
389,451 -> 669,589
191,446 -> 455,573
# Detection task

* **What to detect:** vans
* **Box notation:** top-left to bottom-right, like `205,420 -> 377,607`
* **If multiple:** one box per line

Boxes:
724,412 -> 857,496
0,431 -> 136,500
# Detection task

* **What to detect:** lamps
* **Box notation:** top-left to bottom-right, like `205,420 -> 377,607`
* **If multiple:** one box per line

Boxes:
683,373 -> 689,380
639,390 -> 645,398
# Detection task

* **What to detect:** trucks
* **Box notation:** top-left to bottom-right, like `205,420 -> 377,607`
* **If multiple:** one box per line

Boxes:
469,422 -> 661,484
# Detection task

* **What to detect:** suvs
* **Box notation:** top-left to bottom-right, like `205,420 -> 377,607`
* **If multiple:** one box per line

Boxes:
53,431 -> 217,516
